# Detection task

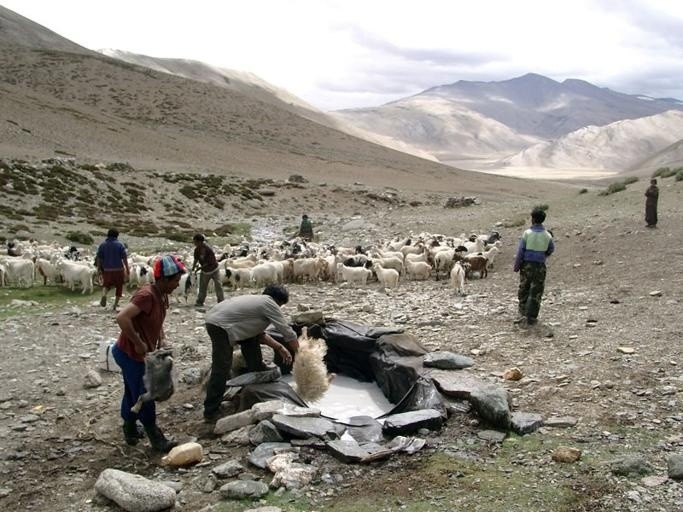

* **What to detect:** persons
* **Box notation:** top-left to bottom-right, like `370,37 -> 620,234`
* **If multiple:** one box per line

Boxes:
299,214 -> 315,243
202,281 -> 300,425
111,254 -> 188,452
95,229 -> 131,312
512,207 -> 556,323
644,178 -> 660,230
191,233 -> 225,307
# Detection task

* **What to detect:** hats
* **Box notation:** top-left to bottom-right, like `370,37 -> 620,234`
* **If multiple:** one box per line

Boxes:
154,255 -> 185,278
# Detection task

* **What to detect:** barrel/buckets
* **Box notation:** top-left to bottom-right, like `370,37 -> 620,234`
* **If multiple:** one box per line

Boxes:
102,339 -> 122,373
162,442 -> 203,466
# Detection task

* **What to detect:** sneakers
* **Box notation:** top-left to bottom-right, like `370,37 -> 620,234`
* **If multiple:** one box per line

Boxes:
513,311 -> 538,324
101,295 -> 107,307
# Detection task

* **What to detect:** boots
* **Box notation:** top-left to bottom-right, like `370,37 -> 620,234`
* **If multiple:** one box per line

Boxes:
145,425 -> 179,454
123,419 -> 144,445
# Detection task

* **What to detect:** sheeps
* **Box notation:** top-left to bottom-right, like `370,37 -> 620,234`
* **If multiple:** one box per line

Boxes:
216,237 -> 338,291
129,344 -> 175,414
337,231 -> 502,293
292,325 -> 336,403
123,246 -> 222,302
0,238 -> 104,295
